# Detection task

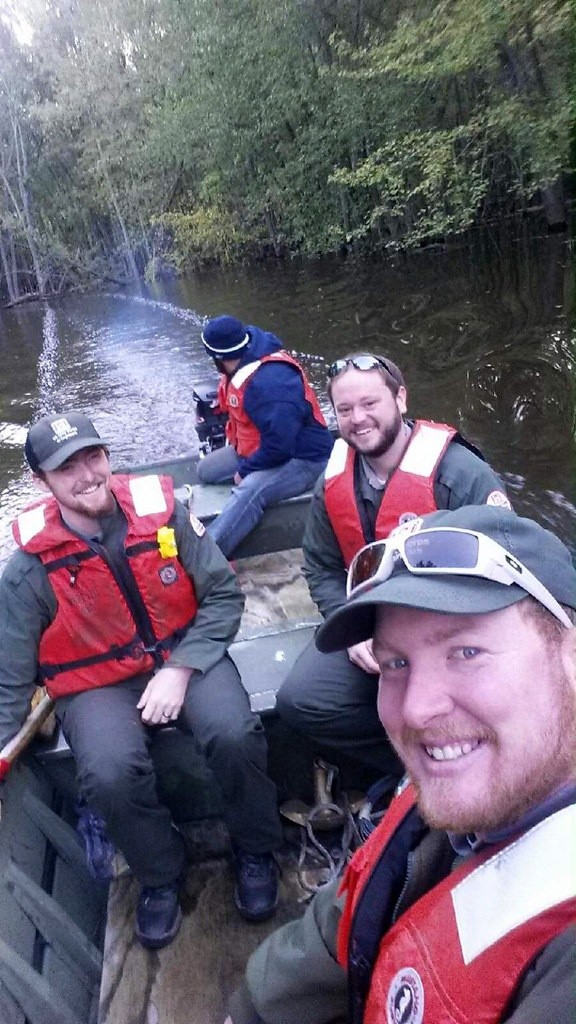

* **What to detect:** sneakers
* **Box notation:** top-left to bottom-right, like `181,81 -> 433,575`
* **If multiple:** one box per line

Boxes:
133,885 -> 182,945
234,851 -> 284,921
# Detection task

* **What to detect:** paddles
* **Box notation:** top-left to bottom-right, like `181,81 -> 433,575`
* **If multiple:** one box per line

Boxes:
1,693 -> 56,782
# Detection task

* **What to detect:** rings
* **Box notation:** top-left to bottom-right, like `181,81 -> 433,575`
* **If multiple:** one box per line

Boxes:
163,711 -> 171,719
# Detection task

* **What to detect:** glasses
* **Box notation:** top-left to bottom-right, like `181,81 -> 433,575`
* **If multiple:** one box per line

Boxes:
347,527 -> 575,631
327,355 -> 394,378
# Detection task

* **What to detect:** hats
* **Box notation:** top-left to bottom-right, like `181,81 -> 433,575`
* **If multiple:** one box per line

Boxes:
316,504 -> 576,653
203,316 -> 250,359
24,413 -> 111,471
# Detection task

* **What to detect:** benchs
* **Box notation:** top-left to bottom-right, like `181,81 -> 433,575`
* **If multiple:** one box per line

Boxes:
174,483 -> 311,560
22,614 -> 325,825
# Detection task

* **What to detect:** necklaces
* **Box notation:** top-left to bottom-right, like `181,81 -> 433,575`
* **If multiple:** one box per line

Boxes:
403,423 -> 408,448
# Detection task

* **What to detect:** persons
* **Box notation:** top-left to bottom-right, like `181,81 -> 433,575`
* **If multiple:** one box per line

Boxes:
0,412 -> 282,948
275,351 -> 516,853
221,504 -> 576,1024
195,315 -> 334,558
